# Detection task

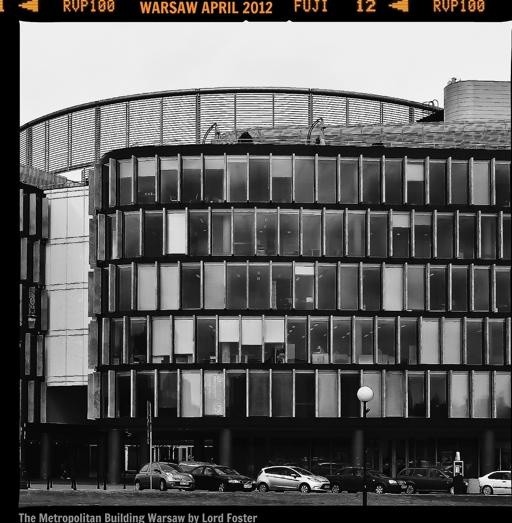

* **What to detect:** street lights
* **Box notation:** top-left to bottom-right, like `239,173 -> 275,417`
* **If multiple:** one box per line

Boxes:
357,387 -> 374,506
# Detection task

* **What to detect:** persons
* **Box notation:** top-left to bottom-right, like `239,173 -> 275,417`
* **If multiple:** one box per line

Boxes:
451,470 -> 466,495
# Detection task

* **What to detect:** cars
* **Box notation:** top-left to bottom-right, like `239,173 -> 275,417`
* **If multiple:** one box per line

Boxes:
135,462 -> 468,495
478,471 -> 512,495
